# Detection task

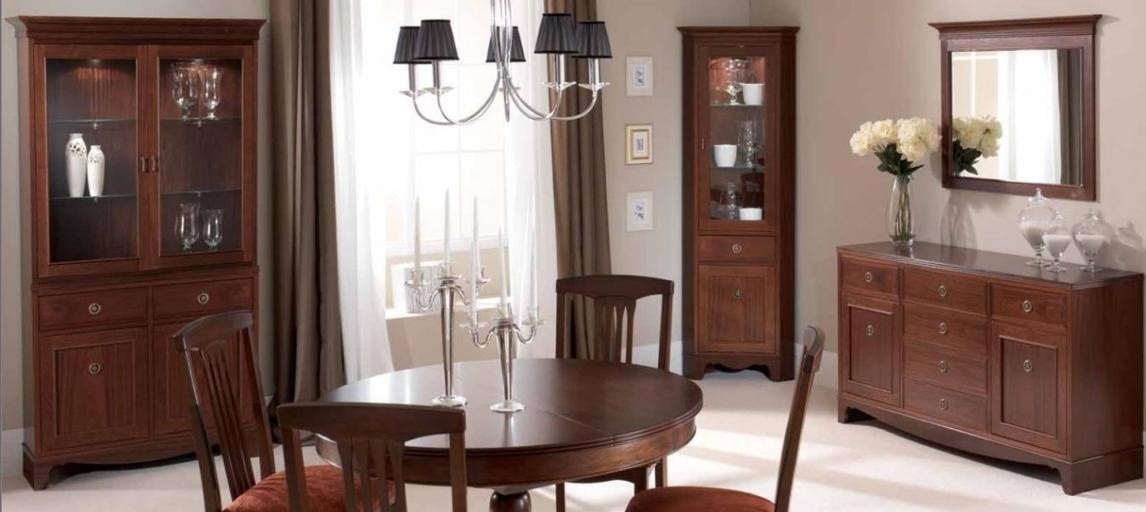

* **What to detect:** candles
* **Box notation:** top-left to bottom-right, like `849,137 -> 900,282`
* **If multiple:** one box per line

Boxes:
411,193 -> 543,328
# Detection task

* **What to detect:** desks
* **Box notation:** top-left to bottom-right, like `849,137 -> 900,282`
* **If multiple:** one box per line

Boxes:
313,357 -> 704,512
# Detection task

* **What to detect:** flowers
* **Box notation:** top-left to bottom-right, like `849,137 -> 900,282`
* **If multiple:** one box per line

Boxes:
849,118 -> 946,247
950,114 -> 1003,177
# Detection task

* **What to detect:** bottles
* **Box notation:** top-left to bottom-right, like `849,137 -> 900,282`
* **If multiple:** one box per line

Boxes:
736,121 -> 756,168
1074,207 -> 1108,274
719,182 -> 737,218
1019,187 -> 1062,267
1041,217 -> 1072,273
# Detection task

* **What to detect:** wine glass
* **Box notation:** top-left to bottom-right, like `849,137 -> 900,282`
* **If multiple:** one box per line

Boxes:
720,63 -> 745,105
173,202 -> 203,254
197,66 -> 224,119
202,209 -> 224,249
170,61 -> 201,119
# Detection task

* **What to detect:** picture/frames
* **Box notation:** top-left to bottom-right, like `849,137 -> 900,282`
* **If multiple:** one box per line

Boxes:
625,122 -> 654,166
624,190 -> 655,233
624,55 -> 655,98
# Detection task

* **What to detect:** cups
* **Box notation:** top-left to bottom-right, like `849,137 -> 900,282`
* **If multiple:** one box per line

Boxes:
742,84 -> 762,106
738,208 -> 763,221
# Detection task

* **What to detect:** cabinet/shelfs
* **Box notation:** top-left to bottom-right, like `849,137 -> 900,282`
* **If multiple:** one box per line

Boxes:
989,271 -> 1145,496
835,242 -> 908,427
676,26 -> 801,382
899,256 -> 991,458
1,14 -> 263,492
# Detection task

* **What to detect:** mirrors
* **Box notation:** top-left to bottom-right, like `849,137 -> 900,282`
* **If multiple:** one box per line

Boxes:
926,14 -> 1106,204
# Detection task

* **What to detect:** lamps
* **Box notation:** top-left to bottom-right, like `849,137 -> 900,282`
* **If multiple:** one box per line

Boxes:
393,1 -> 614,129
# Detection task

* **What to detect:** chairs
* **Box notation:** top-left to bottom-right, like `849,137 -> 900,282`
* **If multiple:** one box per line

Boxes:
171,308 -> 402,511
553,274 -> 678,511
274,400 -> 469,511
625,325 -> 826,511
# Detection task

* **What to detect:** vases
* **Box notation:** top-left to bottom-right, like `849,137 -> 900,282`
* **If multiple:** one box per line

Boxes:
741,82 -> 766,106
739,207 -> 763,223
886,172 -> 917,249
